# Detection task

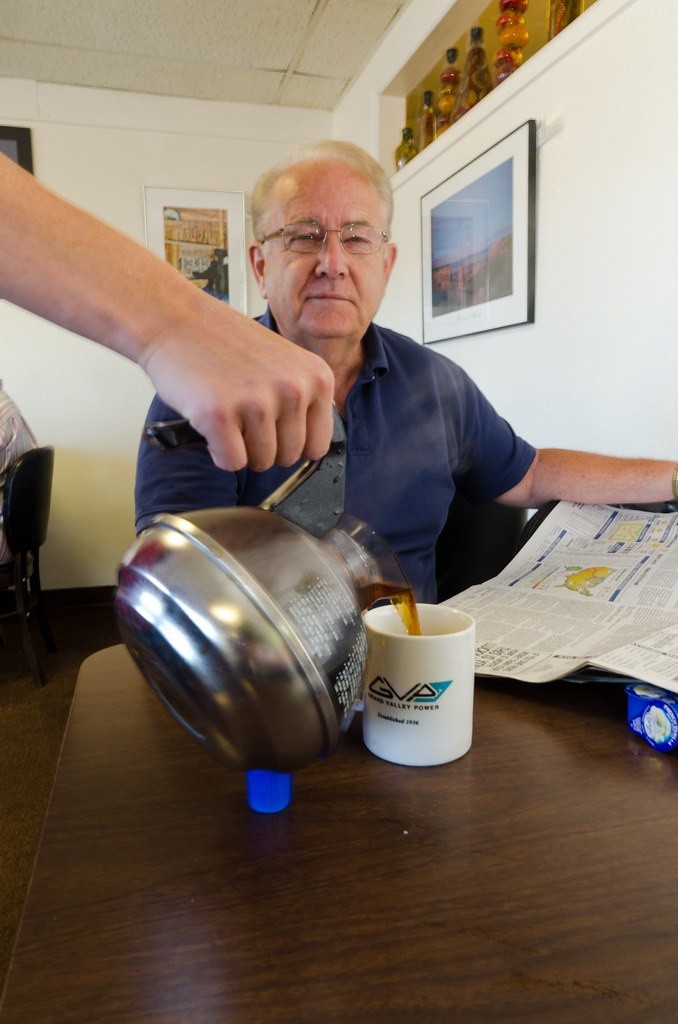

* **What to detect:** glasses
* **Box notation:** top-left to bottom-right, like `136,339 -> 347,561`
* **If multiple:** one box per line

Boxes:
259,221 -> 389,255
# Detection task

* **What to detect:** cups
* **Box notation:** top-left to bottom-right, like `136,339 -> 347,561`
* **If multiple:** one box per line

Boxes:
351,602 -> 476,767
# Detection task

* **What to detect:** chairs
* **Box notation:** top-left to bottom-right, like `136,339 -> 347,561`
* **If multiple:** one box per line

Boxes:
0,444 -> 56,690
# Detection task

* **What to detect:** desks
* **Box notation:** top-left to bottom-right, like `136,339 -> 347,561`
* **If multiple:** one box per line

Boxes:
0,639 -> 678,1024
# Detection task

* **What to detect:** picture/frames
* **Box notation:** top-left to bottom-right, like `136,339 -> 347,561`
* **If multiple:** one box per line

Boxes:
143,184 -> 248,318
0,125 -> 34,176
420,120 -> 536,345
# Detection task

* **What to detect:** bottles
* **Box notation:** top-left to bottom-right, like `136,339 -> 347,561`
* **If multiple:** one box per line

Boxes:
419,91 -> 437,152
549,1 -> 583,42
450,26 -> 495,123
395,127 -> 419,172
494,0 -> 530,85
435,47 -> 465,139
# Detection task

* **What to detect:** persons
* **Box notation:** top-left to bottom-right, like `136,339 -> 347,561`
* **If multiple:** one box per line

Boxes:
134,140 -> 678,613
0,381 -> 39,565
0,147 -> 334,473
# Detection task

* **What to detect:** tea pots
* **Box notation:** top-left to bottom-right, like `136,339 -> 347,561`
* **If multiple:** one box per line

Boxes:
116,397 -> 411,769
